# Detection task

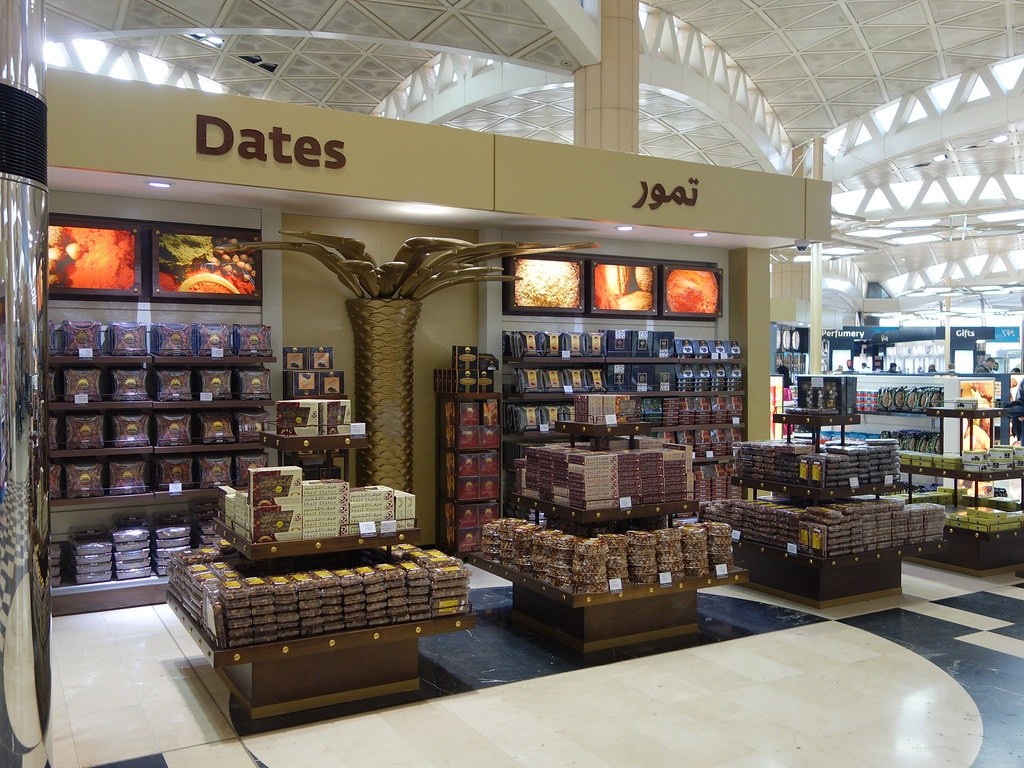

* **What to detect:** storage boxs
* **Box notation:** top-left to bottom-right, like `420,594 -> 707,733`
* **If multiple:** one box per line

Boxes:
435,346 -> 501,553
513,394 -> 740,509
898,399 -> 1023,532
276,345 -> 367,436
797,375 -> 857,413
216,466 -> 417,540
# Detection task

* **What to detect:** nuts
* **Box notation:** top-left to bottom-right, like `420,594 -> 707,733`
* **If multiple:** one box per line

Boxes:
605,264 -> 653,311
209,237 -> 256,282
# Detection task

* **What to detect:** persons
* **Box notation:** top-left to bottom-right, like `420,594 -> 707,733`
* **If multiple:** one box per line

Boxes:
847,360 -> 855,370
1010,367 -> 1020,373
862,362 -> 870,371
974,358 -> 993,373
990,360 -> 995,371
948,363 -> 956,373
1015,378 -> 1024,445
777,365 -> 794,389
928,364 -> 937,372
887,363 -> 897,373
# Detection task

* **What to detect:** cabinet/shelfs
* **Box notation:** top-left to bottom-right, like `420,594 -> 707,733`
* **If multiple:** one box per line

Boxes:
884,407 -> 1024,576
165,515 -> 479,719
467,421 -> 746,654
260,428 -> 369,484
696,414 -> 949,607
498,354 -> 746,461
45,351 -> 277,617
434,391 -> 502,560
